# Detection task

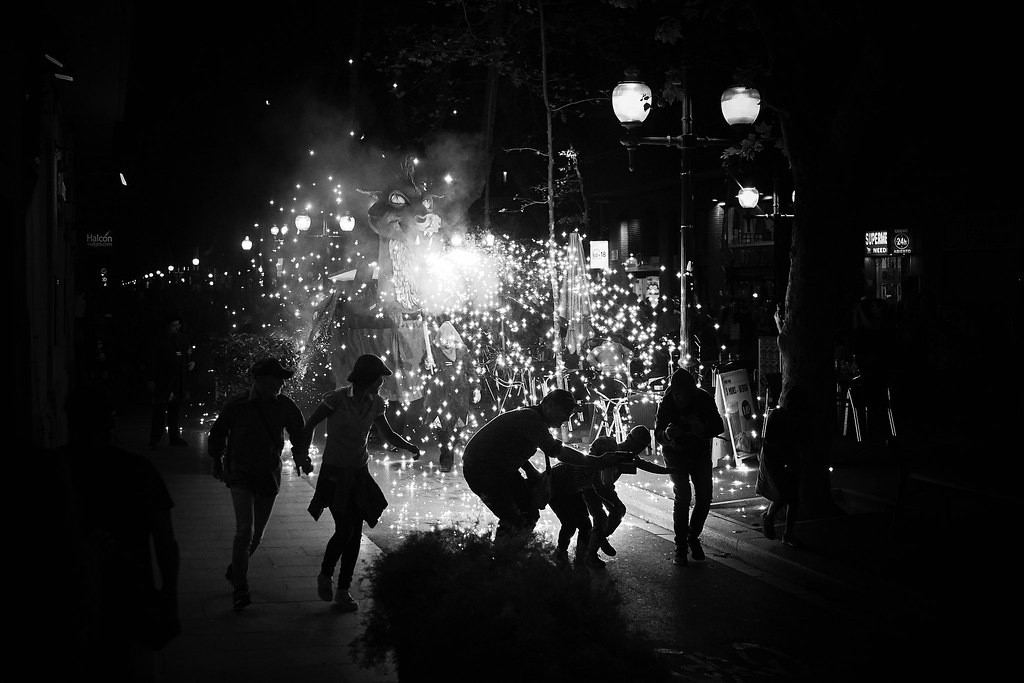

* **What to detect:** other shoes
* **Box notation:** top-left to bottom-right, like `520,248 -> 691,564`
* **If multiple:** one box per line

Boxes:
233,592 -> 252,611
334,589 -> 359,611
781,534 -> 804,546
225,564 -> 238,586
672,550 -> 688,565
592,527 -> 616,556
550,550 -> 573,574
688,536 -> 706,561
317,573 -> 334,602
759,511 -> 777,540
587,554 -> 606,568
574,562 -> 589,577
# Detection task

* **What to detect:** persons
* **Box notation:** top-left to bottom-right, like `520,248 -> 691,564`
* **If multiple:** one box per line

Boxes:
206,356 -> 313,609
654,369 -> 725,564
18,381 -> 182,683
459,390 -> 672,568
146,314 -> 196,448
757,386 -> 812,549
293,355 -> 420,612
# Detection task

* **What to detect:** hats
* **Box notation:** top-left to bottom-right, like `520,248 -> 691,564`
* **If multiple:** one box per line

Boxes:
354,354 -> 393,376
630,425 -> 652,450
251,358 -> 294,379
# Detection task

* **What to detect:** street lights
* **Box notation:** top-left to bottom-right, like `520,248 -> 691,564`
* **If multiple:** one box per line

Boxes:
610,59 -> 764,375
